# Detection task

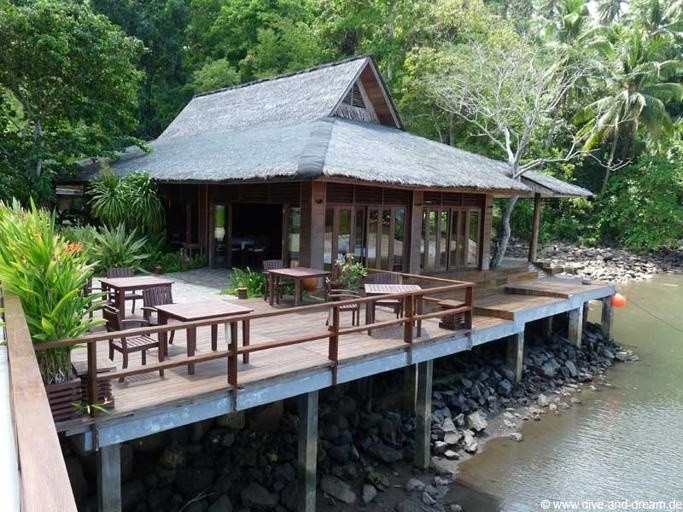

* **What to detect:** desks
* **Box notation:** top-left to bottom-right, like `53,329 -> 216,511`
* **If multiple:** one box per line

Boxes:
97,266 -> 426,383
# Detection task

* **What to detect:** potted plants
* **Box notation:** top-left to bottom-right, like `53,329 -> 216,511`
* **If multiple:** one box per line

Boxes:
0,194 -> 115,423
81,219 -> 152,279
